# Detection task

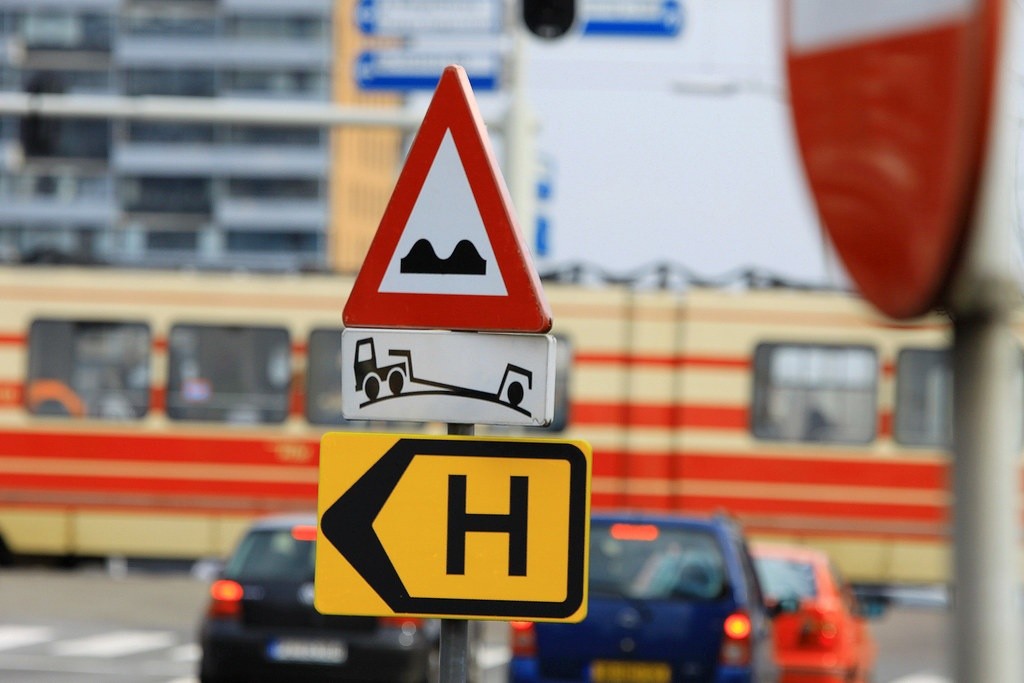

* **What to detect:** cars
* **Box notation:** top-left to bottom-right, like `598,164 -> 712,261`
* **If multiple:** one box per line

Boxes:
745,540 -> 895,683
506,509 -> 799,683
194,512 -> 441,683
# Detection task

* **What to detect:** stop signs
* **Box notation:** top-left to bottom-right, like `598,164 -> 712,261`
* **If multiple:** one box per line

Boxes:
781,0 -> 1009,308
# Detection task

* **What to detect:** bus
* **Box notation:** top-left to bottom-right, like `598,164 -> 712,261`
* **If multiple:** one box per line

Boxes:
0,256 -> 956,604
